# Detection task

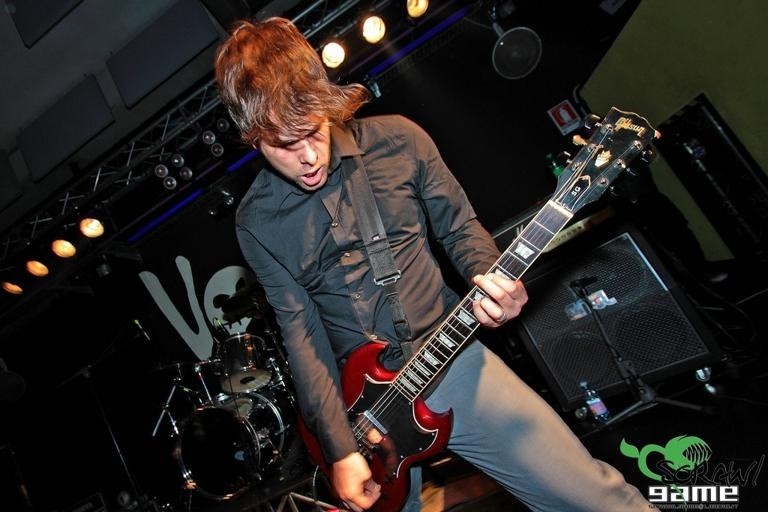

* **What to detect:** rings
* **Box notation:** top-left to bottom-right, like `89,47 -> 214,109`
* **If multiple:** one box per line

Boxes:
497,313 -> 509,323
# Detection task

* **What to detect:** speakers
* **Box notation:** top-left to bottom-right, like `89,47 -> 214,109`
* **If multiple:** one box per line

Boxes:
510,217 -> 719,413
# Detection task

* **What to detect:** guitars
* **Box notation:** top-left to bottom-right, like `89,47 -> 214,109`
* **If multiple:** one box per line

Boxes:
294,105 -> 661,512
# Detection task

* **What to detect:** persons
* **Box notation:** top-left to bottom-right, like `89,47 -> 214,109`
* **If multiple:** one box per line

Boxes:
215,15 -> 660,512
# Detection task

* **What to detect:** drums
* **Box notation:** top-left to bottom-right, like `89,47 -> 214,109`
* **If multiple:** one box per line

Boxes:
176,392 -> 284,502
215,334 -> 272,395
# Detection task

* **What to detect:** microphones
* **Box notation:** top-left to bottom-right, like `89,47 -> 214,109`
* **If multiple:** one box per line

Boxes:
216,319 -> 229,335
133,318 -> 151,342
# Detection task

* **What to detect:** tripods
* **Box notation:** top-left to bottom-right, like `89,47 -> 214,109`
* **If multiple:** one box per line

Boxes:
568,276 -> 720,440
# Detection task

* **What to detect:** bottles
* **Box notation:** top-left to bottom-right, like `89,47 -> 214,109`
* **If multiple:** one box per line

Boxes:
545,154 -> 565,178
580,382 -> 611,423
